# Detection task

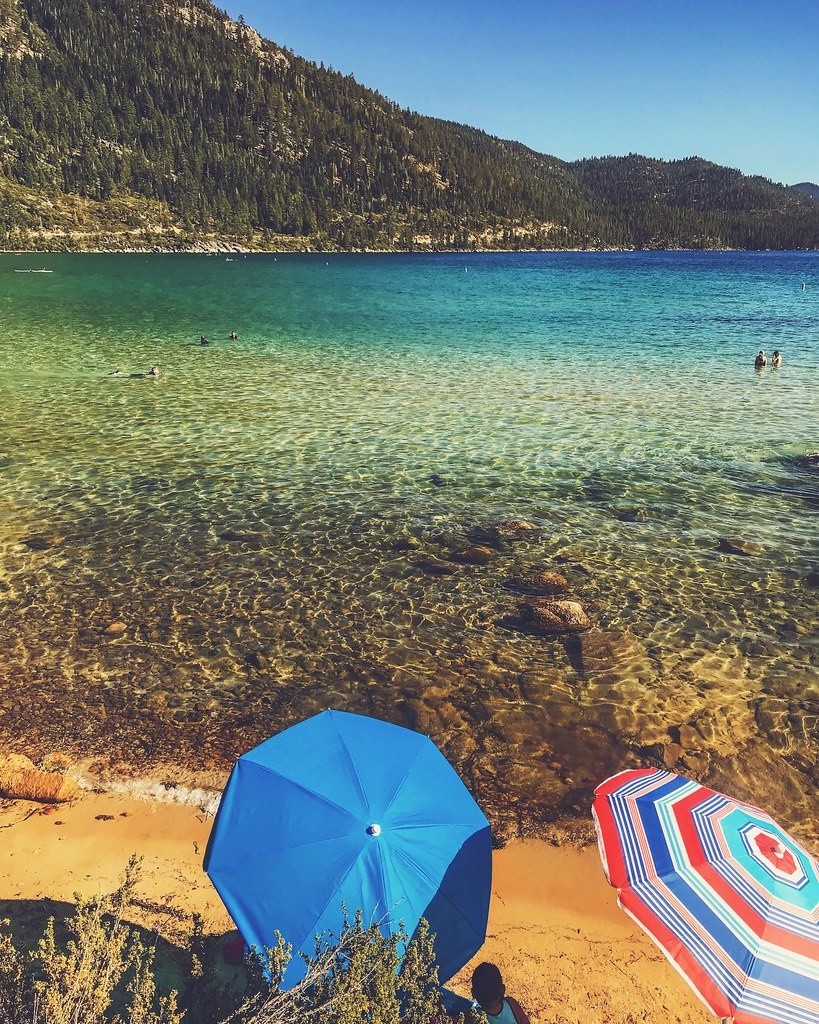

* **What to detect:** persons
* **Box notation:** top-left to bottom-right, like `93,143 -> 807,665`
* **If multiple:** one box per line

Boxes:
771,351 -> 782,364
755,350 -> 767,366
471,962 -> 532,1024
153,367 -> 157,374
201,336 -> 205,341
229,331 -> 238,337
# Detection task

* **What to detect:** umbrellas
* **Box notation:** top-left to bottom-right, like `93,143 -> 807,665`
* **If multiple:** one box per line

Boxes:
591,766 -> 819,1024
205,710 -> 494,1007
